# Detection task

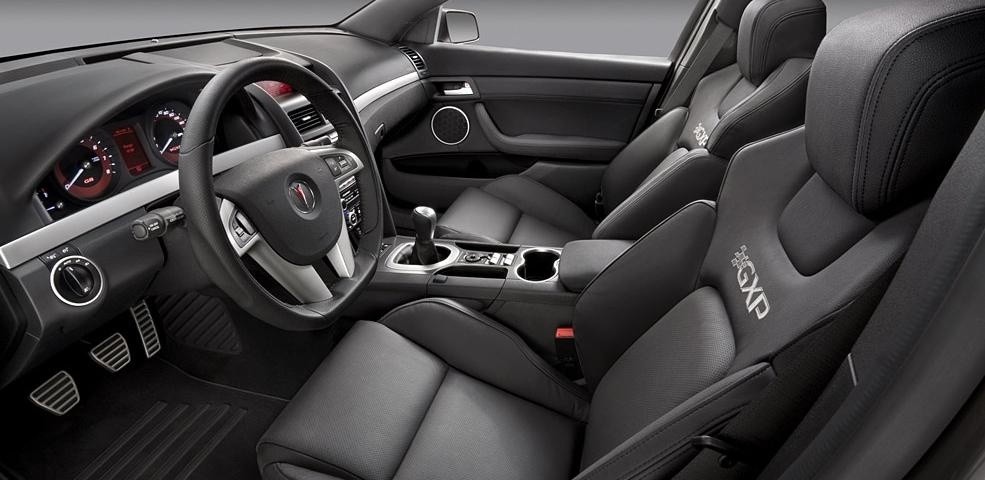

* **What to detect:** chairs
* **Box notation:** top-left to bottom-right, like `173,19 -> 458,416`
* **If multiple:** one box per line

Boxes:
253,0 -> 983,480
